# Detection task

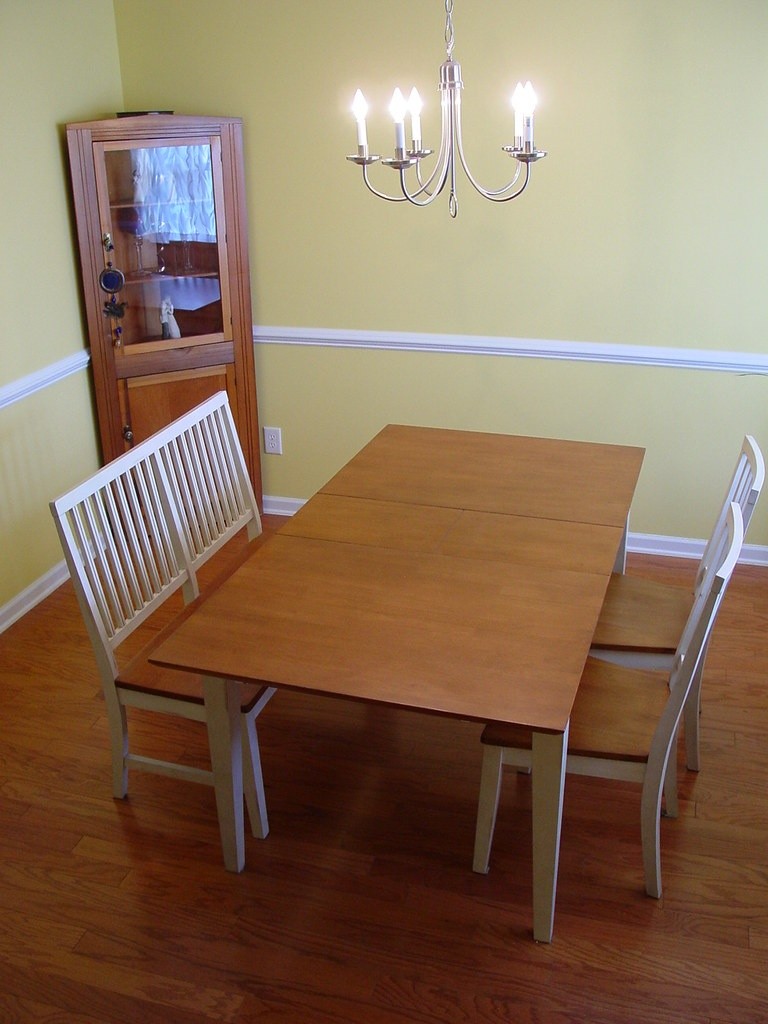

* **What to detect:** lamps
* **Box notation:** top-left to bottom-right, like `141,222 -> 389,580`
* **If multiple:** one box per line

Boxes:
346,0 -> 547,219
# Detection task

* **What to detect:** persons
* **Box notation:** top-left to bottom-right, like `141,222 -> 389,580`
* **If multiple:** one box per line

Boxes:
159,295 -> 181,339
131,169 -> 145,204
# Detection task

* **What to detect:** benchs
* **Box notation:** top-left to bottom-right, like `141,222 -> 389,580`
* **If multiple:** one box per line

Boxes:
50,390 -> 278,841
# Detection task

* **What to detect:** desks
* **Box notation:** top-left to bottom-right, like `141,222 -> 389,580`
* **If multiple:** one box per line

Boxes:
147,423 -> 647,943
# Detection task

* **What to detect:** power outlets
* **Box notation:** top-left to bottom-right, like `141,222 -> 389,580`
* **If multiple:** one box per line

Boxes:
263,427 -> 282,455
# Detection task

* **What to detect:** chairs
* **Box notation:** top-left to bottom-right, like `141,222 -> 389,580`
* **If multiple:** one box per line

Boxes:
588,433 -> 765,773
473,502 -> 745,899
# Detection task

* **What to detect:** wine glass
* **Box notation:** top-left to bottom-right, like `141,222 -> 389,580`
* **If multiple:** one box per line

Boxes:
175,241 -> 201,274
121,206 -> 152,276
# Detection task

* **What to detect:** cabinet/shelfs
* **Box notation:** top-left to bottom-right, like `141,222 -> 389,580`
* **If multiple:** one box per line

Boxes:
65,114 -> 264,533
